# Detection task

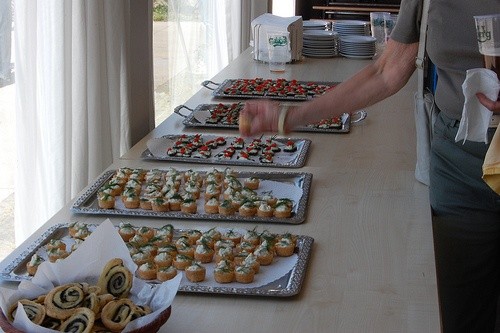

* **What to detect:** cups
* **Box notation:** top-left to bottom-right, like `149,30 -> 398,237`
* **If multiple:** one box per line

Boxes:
370,11 -> 390,60
383,15 -> 398,43
266,31 -> 290,73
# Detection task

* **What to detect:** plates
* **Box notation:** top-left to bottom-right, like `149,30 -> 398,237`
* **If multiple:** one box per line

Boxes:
302,20 -> 377,60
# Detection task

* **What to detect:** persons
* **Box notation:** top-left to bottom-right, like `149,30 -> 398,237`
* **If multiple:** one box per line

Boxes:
238,0 -> 500,333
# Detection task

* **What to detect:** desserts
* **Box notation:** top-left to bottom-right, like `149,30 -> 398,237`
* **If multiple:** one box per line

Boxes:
26,221 -> 295,284
205,101 -> 342,130
7,258 -> 149,333
97,166 -> 296,218
223,77 -> 335,98
166,133 -> 297,163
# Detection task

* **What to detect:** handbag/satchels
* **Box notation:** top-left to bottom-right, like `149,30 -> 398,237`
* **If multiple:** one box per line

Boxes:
413,89 -> 440,187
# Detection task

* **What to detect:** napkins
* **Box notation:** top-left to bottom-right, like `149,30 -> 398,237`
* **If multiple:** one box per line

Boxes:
454,68 -> 500,145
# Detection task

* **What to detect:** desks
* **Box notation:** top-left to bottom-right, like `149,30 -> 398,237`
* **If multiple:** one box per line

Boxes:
0,45 -> 442,333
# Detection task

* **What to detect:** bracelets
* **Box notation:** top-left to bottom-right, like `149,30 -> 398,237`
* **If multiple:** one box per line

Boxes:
276,103 -> 289,137
